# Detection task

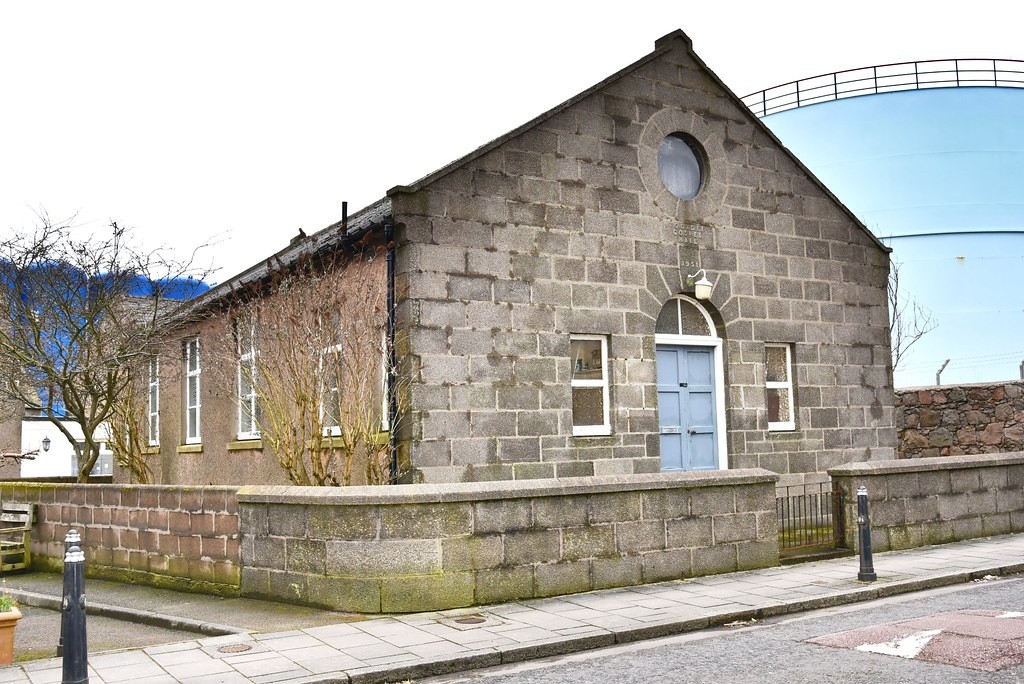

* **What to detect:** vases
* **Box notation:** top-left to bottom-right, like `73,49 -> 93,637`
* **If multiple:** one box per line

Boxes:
0,605 -> 22,666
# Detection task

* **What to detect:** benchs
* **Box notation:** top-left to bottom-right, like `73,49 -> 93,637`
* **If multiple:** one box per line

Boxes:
0,501 -> 34,573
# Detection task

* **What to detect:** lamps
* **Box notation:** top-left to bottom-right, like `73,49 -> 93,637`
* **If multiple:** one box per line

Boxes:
687,269 -> 713,300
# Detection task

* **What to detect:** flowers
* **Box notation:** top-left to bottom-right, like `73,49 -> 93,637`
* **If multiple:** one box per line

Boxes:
0,579 -> 23,615
